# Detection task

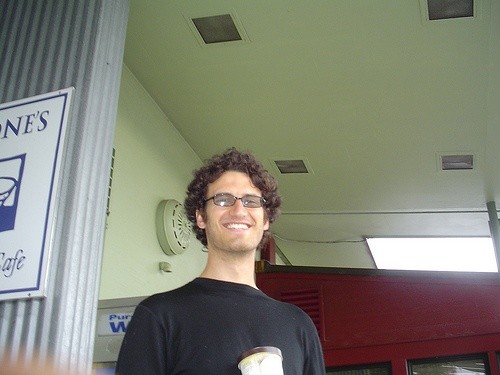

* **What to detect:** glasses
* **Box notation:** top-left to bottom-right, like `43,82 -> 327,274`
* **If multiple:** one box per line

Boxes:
202,193 -> 267,208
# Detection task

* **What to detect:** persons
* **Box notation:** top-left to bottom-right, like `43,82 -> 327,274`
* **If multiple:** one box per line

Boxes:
114,146 -> 326,375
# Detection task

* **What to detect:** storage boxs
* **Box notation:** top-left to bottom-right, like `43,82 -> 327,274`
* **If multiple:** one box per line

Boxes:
91,295 -> 151,362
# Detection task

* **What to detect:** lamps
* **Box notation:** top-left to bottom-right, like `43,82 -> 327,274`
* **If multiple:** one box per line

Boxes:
156,199 -> 193,256
